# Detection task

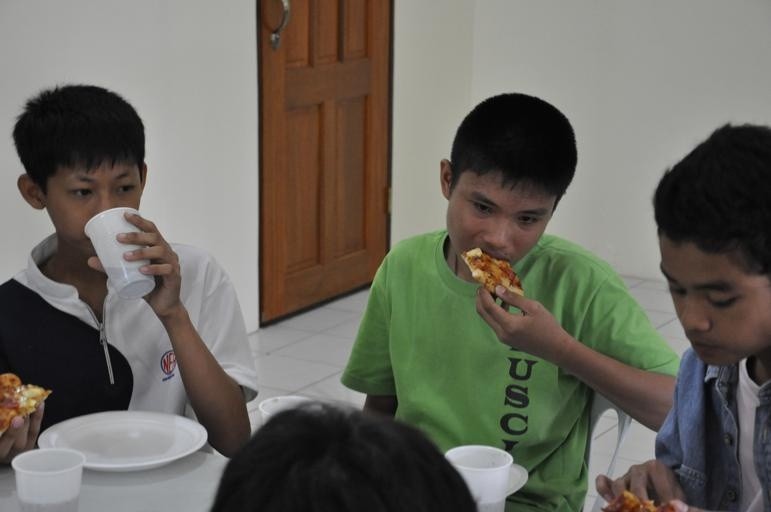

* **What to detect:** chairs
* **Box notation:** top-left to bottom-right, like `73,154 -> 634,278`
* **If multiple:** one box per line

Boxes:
583,392 -> 633,512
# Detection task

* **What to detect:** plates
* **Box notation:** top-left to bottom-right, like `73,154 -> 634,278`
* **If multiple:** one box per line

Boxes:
505,464 -> 529,498
37,410 -> 208,473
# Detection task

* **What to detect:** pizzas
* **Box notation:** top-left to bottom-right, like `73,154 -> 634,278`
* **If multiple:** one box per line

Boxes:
601,491 -> 673,512
461,247 -> 525,297
0,371 -> 52,436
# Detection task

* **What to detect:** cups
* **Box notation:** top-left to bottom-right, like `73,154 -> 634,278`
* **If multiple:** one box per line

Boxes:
259,395 -> 311,427
83,207 -> 156,302
445,443 -> 514,512
10,448 -> 86,512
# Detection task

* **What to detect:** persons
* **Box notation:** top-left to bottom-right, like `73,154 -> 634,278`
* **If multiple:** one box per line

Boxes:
212,397 -> 474,508
0,81 -> 261,461
339,90 -> 682,511
595,119 -> 771,512
